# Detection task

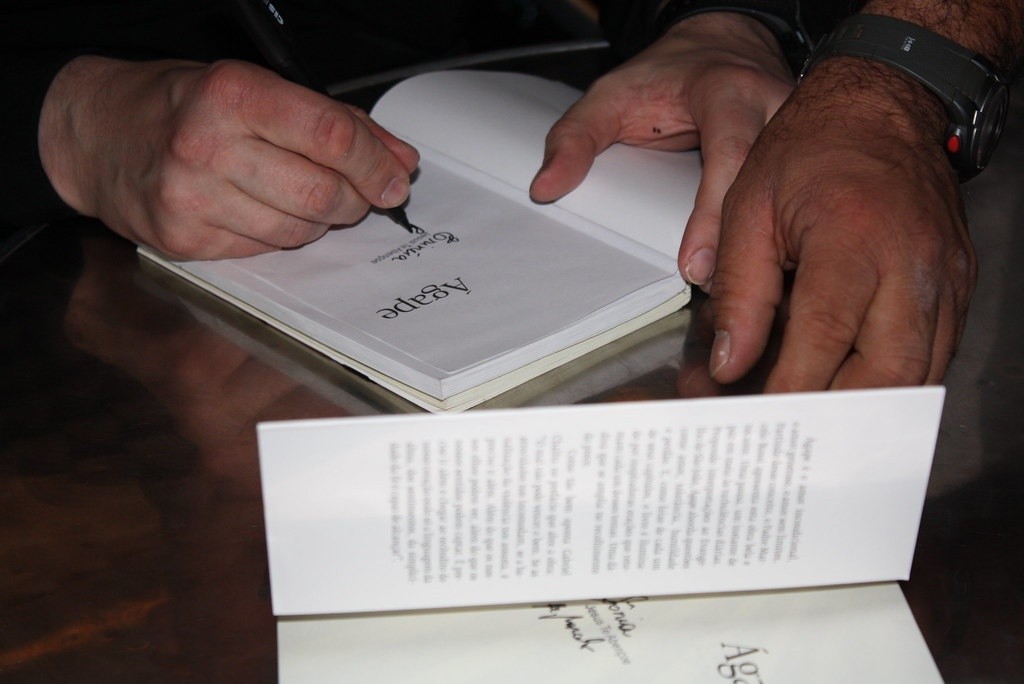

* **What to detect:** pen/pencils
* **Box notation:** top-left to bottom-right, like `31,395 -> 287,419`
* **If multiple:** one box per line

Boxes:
224,0 -> 413,235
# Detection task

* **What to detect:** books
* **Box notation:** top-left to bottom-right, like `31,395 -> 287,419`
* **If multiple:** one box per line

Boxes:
137,67 -> 701,417
254,385 -> 946,684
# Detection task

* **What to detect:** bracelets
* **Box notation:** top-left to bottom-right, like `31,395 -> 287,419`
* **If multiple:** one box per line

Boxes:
655,0 -> 814,78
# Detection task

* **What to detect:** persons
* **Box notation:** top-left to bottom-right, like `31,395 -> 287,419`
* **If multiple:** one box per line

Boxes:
710,0 -> 1024,392
0,0 -> 812,297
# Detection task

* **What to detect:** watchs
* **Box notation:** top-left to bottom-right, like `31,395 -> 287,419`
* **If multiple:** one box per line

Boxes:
798,11 -> 1011,184
